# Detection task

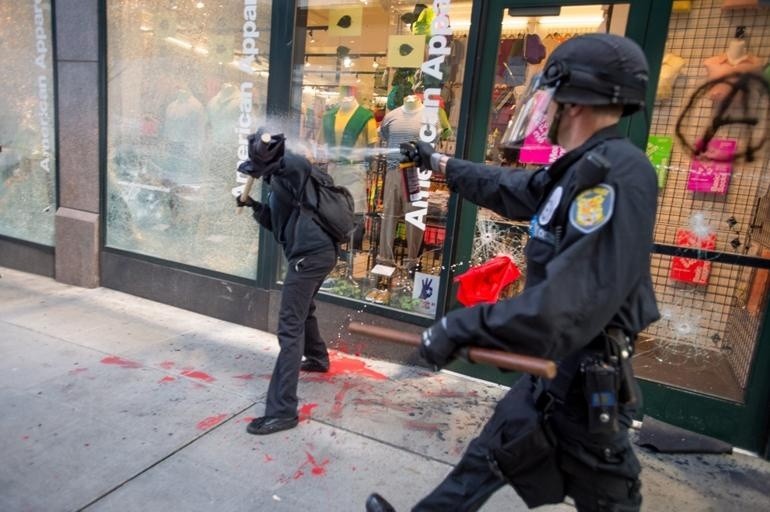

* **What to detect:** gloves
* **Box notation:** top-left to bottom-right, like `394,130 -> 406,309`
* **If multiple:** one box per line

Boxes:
235,194 -> 257,208
399,140 -> 444,174
419,317 -> 461,373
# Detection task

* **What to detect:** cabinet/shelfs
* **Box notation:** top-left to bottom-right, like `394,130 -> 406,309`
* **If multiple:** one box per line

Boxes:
365,137 -> 457,278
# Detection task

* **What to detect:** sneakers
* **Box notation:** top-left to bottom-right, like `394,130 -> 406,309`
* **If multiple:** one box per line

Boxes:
246,414 -> 299,435
300,358 -> 330,372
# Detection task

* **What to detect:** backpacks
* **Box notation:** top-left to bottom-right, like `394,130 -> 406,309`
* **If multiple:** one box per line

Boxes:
310,164 -> 355,246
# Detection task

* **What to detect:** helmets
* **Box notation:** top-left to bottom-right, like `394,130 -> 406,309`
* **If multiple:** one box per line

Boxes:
537,32 -> 652,118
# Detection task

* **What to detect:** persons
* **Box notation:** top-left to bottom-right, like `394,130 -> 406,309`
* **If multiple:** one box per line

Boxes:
162,88 -> 204,156
236,127 -> 338,436
205,80 -> 247,173
701,41 -> 764,103
317,95 -> 379,215
366,32 -> 661,512
654,42 -> 686,102
378,94 -> 442,269
